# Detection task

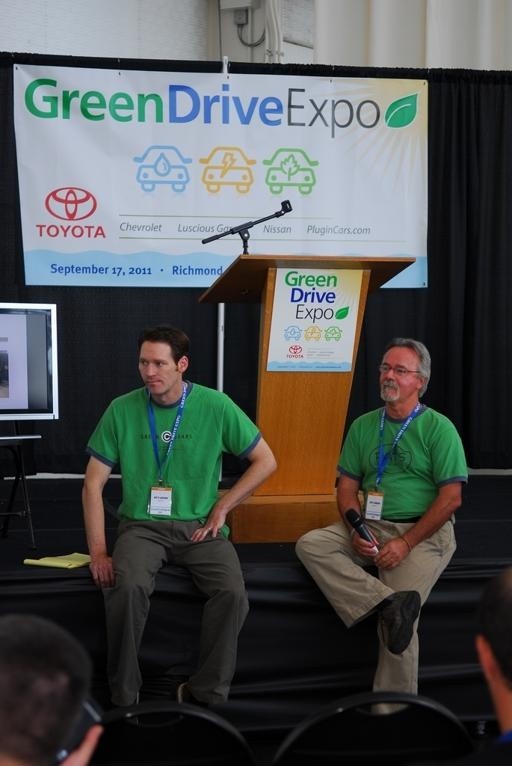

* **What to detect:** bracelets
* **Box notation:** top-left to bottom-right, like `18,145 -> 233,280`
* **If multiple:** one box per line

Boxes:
400,535 -> 413,553
347,526 -> 357,539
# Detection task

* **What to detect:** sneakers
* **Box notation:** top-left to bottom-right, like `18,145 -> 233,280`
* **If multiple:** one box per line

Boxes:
378,590 -> 422,656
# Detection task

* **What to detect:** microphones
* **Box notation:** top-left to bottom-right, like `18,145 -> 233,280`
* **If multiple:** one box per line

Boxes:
345,508 -> 381,555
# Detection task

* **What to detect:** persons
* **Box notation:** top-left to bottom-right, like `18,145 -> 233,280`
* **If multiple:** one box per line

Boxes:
461,565 -> 512,766
293,335 -> 469,719
80,324 -> 280,713
0,610 -> 104,766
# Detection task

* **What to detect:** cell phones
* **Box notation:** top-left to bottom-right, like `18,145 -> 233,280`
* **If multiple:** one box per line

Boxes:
56,703 -> 102,761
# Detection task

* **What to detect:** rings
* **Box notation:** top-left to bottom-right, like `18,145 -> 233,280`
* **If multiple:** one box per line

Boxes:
206,527 -> 211,531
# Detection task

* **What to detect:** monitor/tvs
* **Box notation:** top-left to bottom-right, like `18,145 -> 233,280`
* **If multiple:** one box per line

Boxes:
0,303 -> 61,439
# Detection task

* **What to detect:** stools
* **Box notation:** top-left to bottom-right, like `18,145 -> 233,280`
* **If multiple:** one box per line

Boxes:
0,435 -> 45,554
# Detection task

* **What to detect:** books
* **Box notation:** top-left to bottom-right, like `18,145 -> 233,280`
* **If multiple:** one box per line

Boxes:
24,551 -> 94,570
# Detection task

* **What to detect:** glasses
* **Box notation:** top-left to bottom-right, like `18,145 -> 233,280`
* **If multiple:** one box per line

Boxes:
379,364 -> 420,376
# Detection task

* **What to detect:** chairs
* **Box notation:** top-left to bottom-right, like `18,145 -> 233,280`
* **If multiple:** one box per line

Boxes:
90,700 -> 259,761
268,690 -> 471,760
